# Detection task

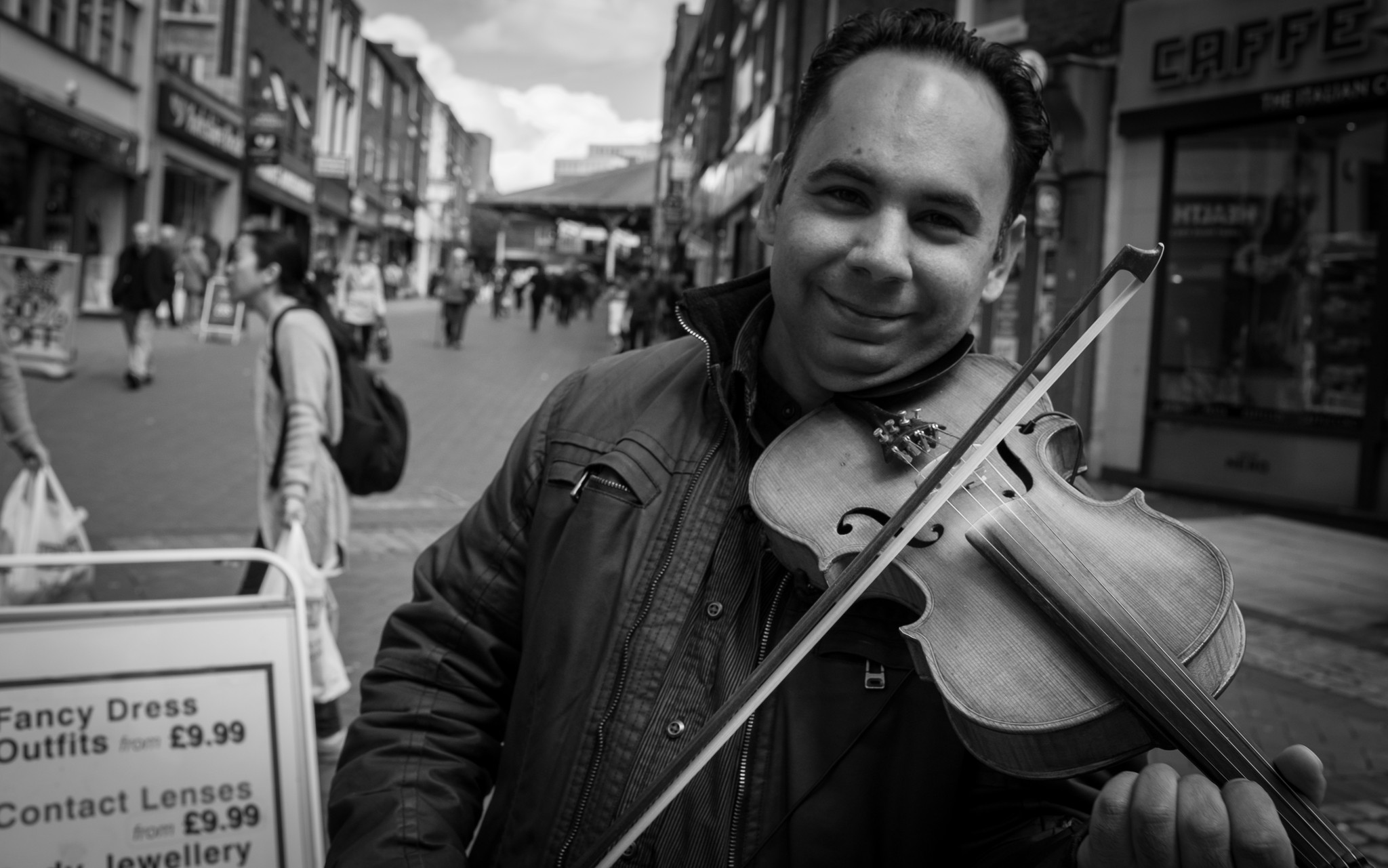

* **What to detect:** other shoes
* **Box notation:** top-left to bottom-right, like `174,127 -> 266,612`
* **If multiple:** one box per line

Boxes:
314,731 -> 345,765
124,370 -> 152,391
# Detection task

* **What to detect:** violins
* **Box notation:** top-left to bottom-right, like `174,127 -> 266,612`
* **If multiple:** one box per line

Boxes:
745,351 -> 1363,868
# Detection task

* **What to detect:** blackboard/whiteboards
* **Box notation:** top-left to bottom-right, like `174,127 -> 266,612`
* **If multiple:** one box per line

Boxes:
199,276 -> 246,333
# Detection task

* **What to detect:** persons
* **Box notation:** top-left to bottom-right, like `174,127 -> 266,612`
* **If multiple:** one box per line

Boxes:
224,227 -> 352,762
0,302 -> 50,474
491,260 -> 654,354
111,220 -> 176,390
322,7 -> 1324,868
334,246 -> 385,361
172,236 -> 212,323
435,247 -> 481,351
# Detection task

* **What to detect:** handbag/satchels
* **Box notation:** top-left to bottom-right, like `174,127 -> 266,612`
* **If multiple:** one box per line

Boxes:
376,325 -> 391,363
0,464 -> 96,608
257,521 -> 351,702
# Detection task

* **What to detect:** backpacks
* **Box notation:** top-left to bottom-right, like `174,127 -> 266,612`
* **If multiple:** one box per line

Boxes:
267,304 -> 409,497
